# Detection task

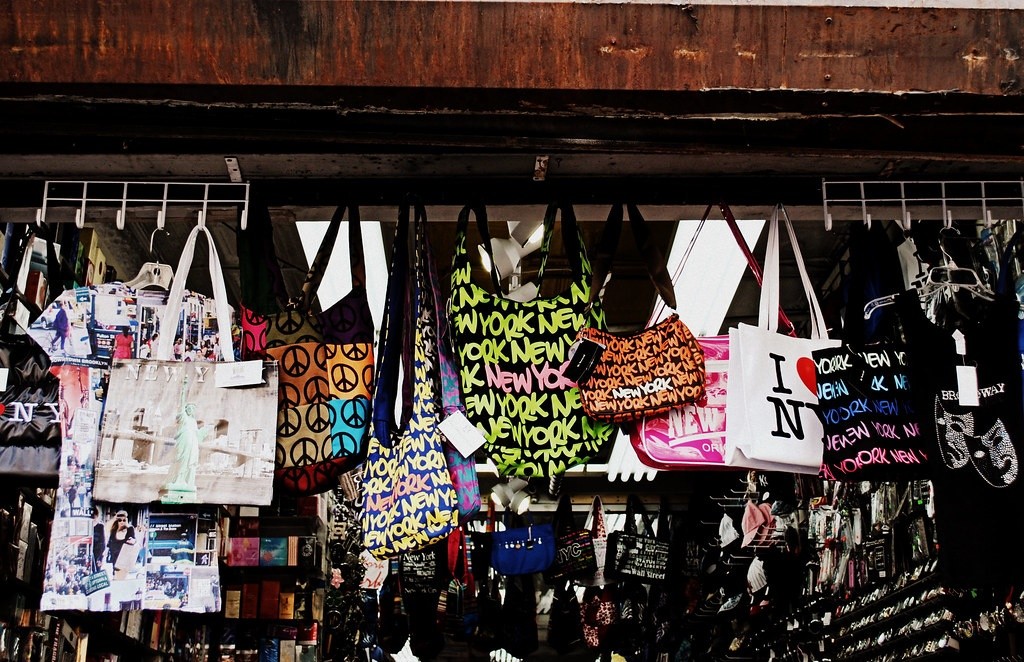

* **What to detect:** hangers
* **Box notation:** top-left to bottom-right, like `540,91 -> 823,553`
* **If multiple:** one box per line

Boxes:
122,228 -> 176,293
864,227 -> 994,320
863,232 -> 993,313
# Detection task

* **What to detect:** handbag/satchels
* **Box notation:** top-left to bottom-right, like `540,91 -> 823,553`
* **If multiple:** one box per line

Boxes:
2,201 -> 943,661
